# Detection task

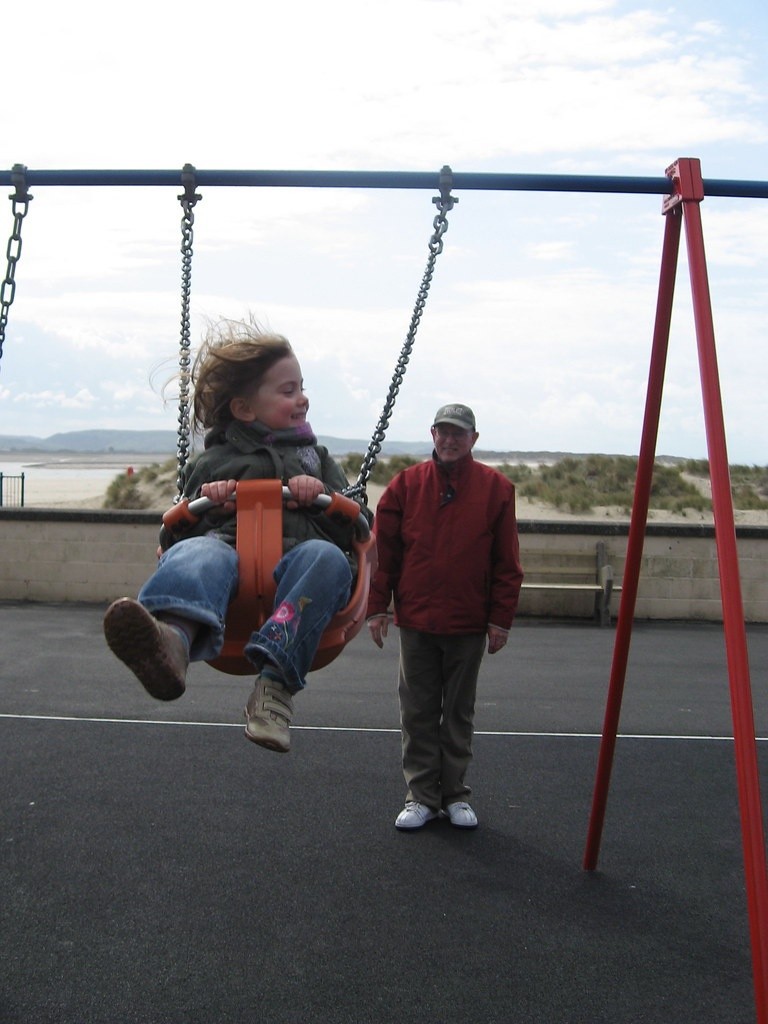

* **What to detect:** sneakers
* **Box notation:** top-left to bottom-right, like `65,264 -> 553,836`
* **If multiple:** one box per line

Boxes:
103,598 -> 188,701
242,675 -> 294,753
395,801 -> 440,831
442,801 -> 478,830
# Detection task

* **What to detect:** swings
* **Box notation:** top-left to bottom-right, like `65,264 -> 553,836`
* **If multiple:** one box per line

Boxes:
0,158 -> 31,364
153,160 -> 460,681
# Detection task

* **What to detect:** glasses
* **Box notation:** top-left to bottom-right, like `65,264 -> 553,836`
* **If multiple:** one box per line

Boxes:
435,429 -> 474,442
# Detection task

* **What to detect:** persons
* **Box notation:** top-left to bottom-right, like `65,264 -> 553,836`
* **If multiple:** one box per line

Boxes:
365,404 -> 525,831
102,336 -> 372,754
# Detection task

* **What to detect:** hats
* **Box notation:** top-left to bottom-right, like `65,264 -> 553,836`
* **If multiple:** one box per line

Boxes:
433,404 -> 475,432
